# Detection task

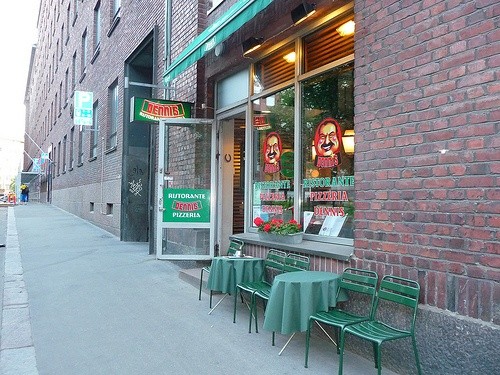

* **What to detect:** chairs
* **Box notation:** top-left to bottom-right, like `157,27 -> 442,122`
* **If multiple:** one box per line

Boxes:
199,238 -> 244,309
233,249 -> 310,347
304,267 -> 422,375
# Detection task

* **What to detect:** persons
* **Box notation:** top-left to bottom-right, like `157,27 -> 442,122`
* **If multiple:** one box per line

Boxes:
20,184 -> 29,202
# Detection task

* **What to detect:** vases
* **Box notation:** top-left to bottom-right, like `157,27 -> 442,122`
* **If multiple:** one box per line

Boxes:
257,230 -> 303,244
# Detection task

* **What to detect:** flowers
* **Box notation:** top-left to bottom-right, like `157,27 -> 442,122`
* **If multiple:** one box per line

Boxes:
254,217 -> 303,237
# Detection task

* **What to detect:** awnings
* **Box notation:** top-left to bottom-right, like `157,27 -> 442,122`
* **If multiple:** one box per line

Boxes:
160,0 -> 309,85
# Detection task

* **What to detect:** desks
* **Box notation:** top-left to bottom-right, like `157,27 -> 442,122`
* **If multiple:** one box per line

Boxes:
205,255 -> 266,320
263,271 -> 349,356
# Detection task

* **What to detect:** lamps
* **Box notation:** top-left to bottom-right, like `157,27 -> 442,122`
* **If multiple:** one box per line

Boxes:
242,36 -> 263,56
291,2 -> 317,26
